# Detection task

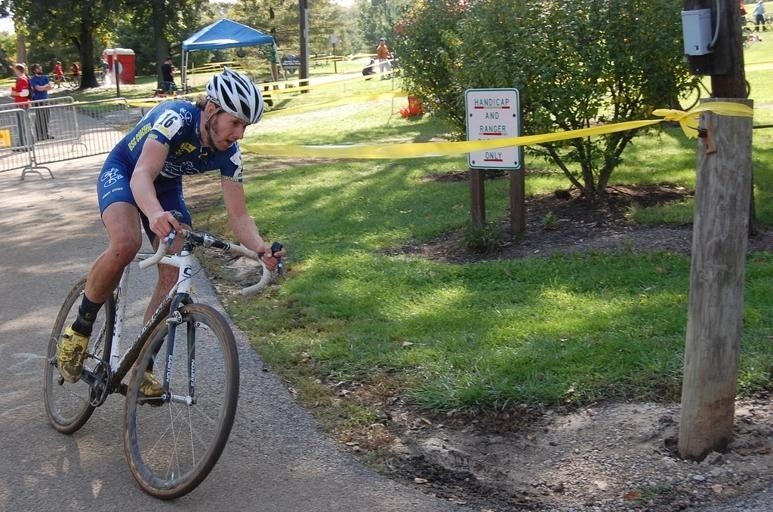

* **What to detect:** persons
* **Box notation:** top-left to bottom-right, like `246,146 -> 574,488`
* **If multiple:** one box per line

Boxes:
8,63 -> 32,153
69,63 -> 80,89
51,60 -> 65,90
375,38 -> 394,81
360,59 -> 377,82
28,64 -> 53,142
52,65 -> 287,405
752,1 -> 768,34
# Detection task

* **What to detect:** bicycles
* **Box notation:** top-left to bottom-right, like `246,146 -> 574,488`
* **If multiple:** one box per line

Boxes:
141,78 -> 199,116
674,66 -> 751,112
741,16 -> 757,36
41,211 -> 286,503
68,77 -> 80,87
760,15 -> 773,32
46,74 -> 73,89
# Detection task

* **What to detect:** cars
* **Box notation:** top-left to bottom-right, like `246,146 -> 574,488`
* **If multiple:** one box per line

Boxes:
275,55 -> 301,74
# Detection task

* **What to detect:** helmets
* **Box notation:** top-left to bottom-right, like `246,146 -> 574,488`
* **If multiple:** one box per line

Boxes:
207,66 -> 264,123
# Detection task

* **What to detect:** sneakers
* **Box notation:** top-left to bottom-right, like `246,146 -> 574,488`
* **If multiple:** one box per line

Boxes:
59,324 -> 89,383
140,371 -> 162,396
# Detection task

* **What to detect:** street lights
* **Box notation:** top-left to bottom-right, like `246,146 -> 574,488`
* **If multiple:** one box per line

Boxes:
330,36 -> 339,72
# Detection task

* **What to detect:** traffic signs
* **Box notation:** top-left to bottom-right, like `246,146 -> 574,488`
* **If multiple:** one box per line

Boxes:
465,88 -> 520,169
